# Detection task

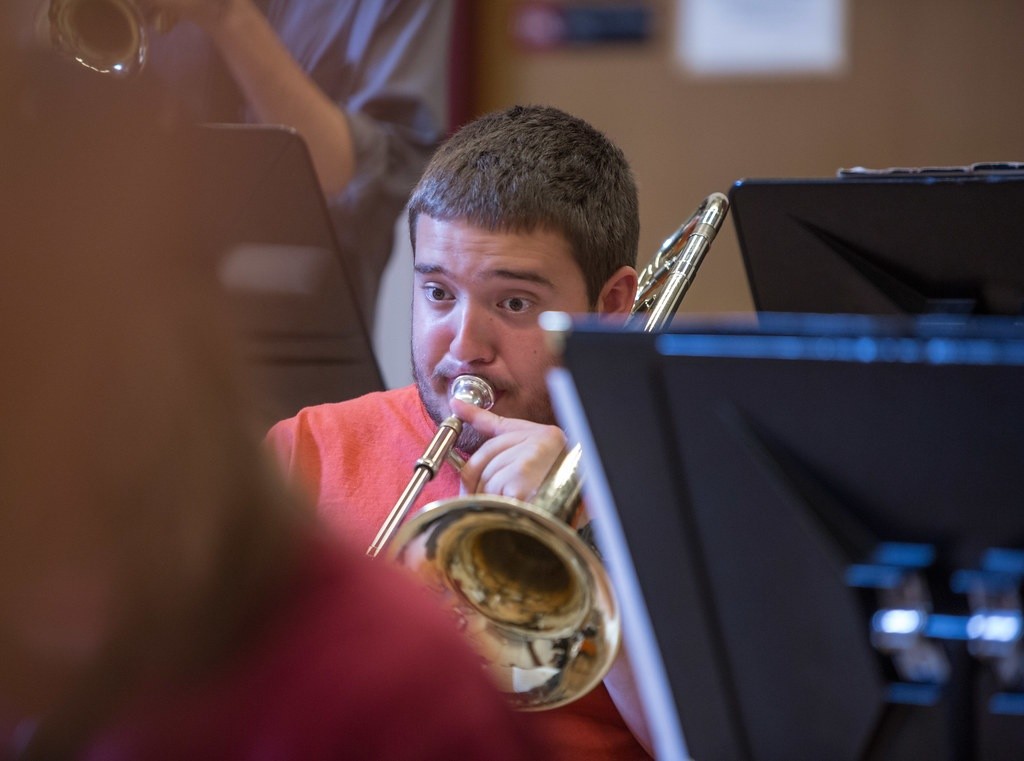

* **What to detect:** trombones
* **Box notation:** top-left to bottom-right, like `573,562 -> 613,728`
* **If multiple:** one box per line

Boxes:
352,189 -> 731,712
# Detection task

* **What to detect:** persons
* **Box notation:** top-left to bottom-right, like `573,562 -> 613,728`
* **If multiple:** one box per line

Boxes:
267,105 -> 656,760
1,0 -> 552,758
186,0 -> 455,288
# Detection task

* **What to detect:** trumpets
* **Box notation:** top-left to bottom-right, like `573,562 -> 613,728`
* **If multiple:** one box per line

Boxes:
47,1 -> 181,88
